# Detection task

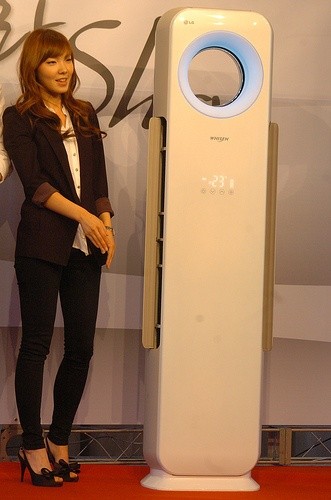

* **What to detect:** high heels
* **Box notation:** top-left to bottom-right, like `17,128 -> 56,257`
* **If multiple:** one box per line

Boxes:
17,437 -> 81,487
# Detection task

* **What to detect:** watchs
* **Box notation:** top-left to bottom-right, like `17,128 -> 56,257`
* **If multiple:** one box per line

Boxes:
105,226 -> 114,236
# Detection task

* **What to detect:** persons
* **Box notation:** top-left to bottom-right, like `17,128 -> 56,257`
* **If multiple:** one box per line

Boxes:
2,28 -> 116,487
0,86 -> 15,184
